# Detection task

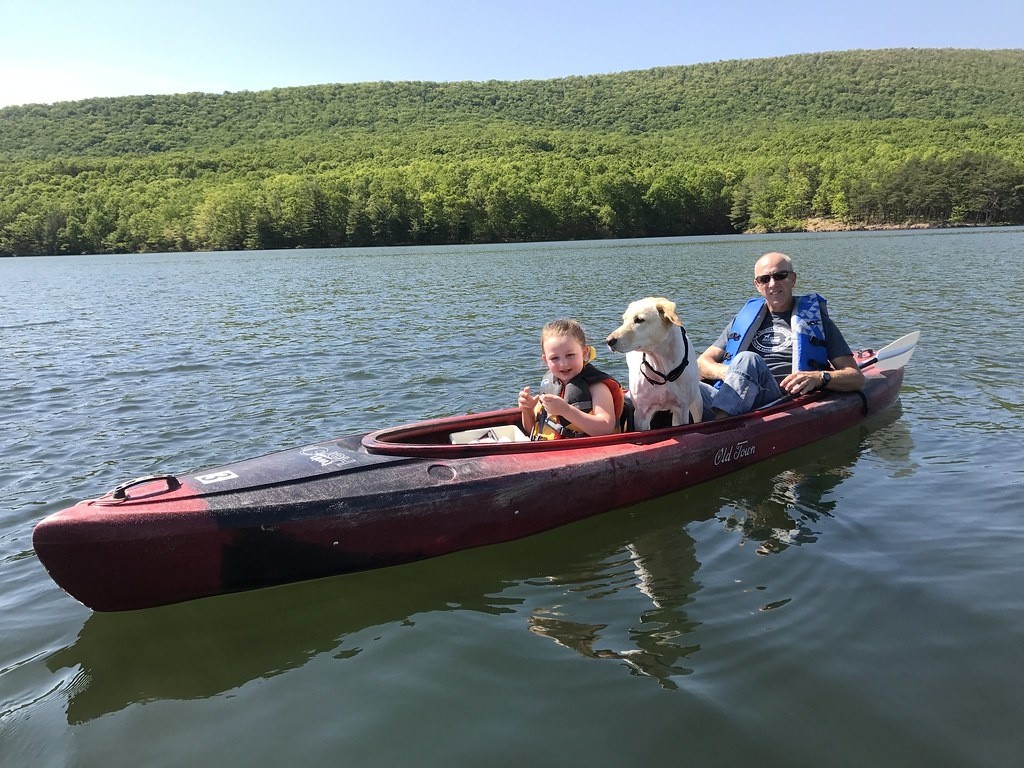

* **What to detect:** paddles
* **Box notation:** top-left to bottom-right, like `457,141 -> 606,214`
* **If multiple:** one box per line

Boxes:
746,329 -> 923,414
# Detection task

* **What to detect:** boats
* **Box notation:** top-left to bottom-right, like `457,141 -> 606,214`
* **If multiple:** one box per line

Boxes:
30,329 -> 904,613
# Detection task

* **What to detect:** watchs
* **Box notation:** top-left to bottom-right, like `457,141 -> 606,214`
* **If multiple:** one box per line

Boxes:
819,370 -> 831,389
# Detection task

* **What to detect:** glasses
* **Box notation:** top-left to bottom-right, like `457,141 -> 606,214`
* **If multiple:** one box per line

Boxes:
756,270 -> 794,283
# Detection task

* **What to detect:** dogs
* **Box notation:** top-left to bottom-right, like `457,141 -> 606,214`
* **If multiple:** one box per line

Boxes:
606,297 -> 703,433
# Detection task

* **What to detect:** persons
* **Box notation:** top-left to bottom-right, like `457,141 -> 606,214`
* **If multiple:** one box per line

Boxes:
696,252 -> 865,421
518,319 -> 617,442
